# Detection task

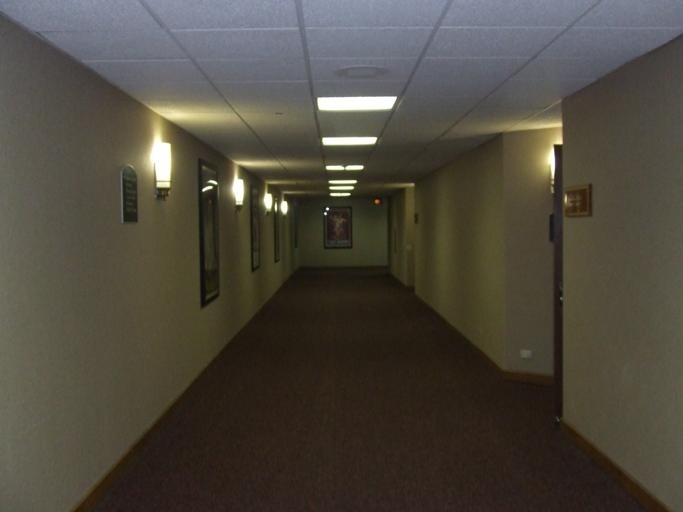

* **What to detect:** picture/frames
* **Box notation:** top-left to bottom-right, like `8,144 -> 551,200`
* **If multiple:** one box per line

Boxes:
322,205 -> 353,250
248,185 -> 262,272
196,154 -> 221,308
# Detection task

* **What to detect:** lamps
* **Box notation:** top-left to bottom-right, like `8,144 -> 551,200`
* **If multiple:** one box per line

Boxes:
153,142 -> 173,200
